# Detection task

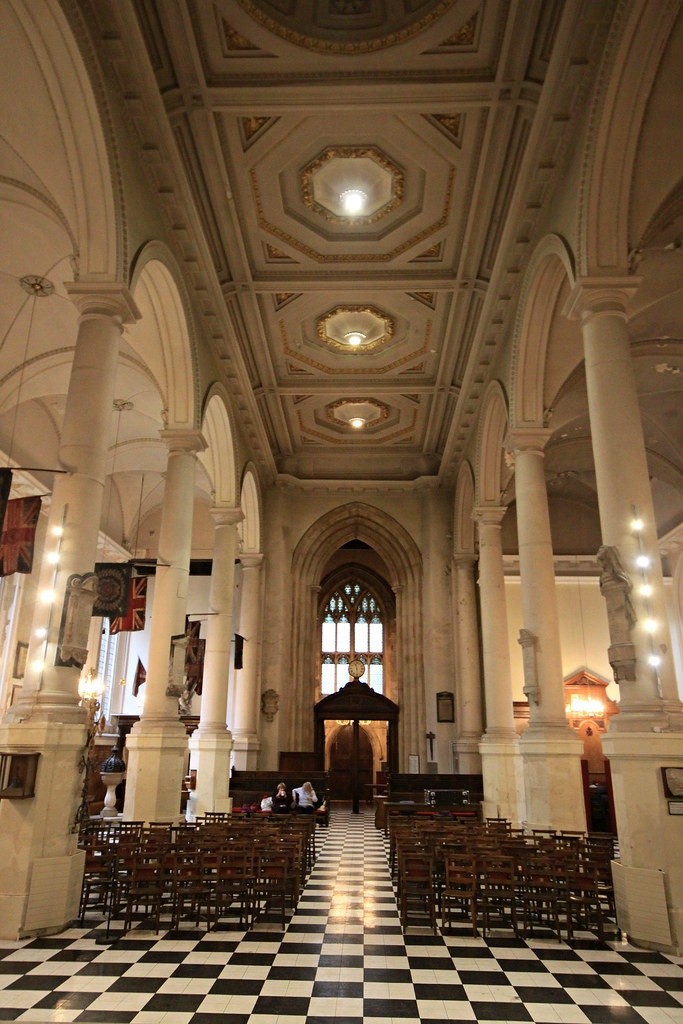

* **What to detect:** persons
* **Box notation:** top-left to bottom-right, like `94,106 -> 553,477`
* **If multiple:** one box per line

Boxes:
272,783 -> 293,814
292,782 -> 318,813
261,792 -> 273,812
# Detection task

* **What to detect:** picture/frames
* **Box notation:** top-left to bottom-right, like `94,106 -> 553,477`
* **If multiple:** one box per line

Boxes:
10,684 -> 23,706
13,642 -> 29,678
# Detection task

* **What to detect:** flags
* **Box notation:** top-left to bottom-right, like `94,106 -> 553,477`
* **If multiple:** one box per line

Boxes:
234,634 -> 244,669
109,576 -> 148,635
133,657 -> 146,696
0,495 -> 42,577
95,563 -> 132,615
186,614 -> 206,695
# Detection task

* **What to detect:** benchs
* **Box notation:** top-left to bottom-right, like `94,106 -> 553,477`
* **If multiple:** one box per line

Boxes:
387,774 -> 484,802
224,766 -> 331,828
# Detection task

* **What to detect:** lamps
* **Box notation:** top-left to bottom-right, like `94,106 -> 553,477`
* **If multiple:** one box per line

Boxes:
344,333 -> 366,347
348,417 -> 366,429
566,558 -> 607,717
80,616 -> 104,694
339,190 -> 370,215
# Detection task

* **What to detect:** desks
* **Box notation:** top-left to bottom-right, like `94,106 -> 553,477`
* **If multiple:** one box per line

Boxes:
384,801 -> 483,835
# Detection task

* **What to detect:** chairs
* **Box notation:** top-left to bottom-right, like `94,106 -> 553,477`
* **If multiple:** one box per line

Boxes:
77,816 -> 319,933
389,812 -> 616,944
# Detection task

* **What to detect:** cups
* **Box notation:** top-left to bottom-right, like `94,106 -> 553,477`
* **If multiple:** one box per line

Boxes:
281,791 -> 285,796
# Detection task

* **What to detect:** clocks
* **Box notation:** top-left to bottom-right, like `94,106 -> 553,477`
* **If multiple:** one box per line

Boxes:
348,659 -> 365,678
